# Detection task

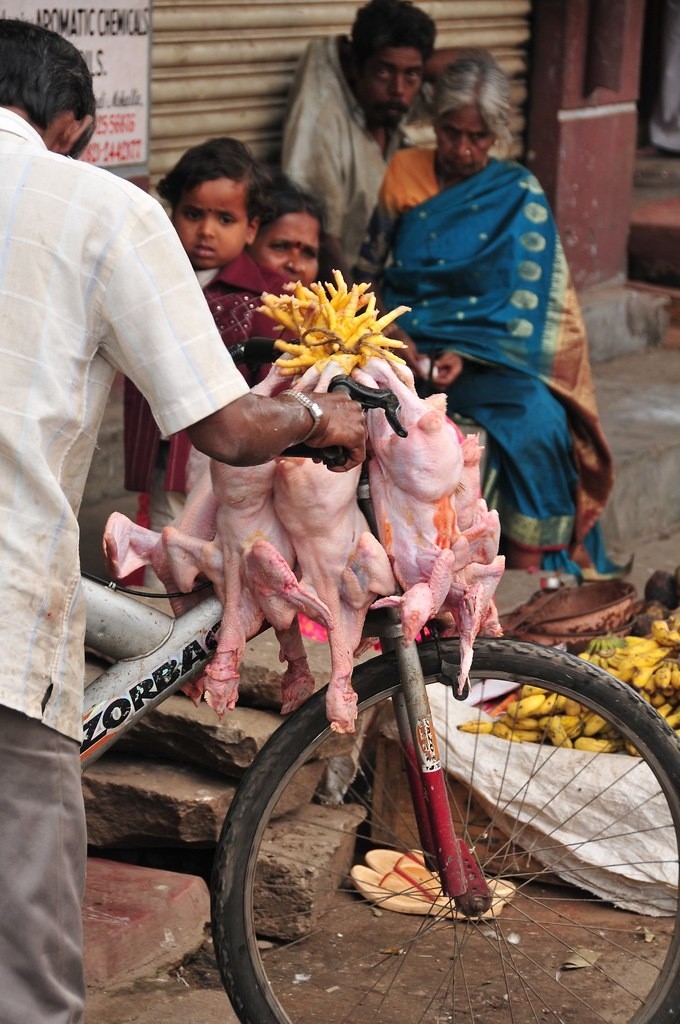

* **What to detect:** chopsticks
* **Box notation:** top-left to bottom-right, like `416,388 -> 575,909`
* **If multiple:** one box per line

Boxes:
501,582 -> 569,631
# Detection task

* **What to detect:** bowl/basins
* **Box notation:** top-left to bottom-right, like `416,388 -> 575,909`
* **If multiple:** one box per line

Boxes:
510,580 -> 640,650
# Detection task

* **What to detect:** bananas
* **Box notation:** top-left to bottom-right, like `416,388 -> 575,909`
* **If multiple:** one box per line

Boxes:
457,606 -> 680,758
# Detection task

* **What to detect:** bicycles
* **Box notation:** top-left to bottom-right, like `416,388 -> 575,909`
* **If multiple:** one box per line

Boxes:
80,372 -> 680,1024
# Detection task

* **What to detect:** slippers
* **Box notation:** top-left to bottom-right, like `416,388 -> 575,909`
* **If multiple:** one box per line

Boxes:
350,849 -> 517,921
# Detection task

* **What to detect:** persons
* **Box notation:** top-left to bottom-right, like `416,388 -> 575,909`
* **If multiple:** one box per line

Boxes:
353,60 -> 634,592
282,0 -> 437,271
1,19 -> 369,1024
156,137 -> 300,388
254,185 -> 346,295
